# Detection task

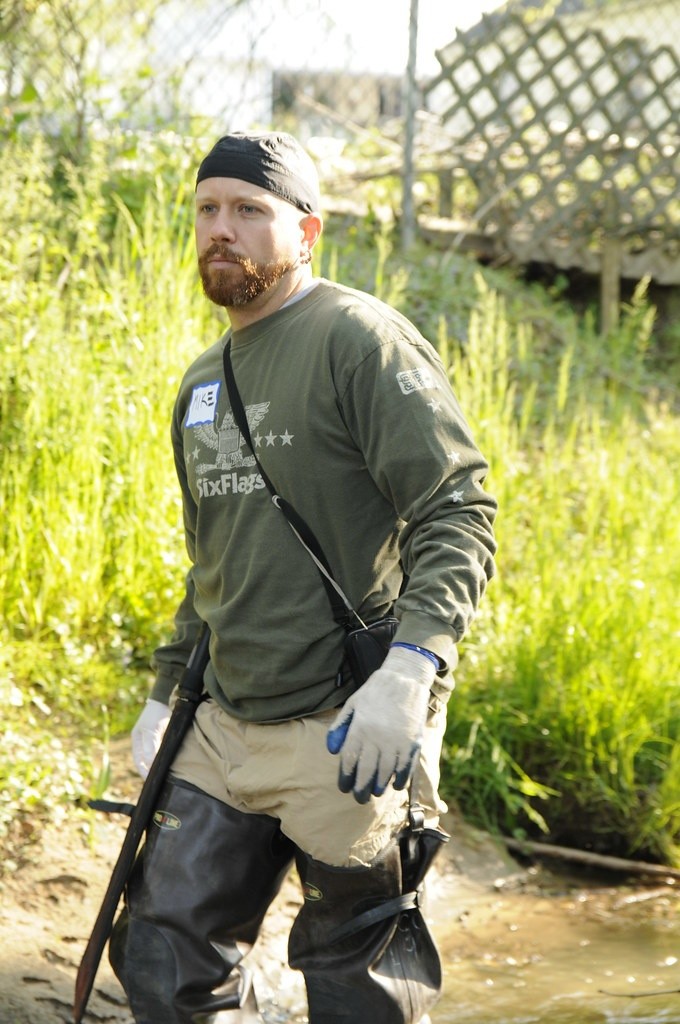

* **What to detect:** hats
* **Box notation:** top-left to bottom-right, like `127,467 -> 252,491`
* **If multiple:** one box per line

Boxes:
195,130 -> 320,214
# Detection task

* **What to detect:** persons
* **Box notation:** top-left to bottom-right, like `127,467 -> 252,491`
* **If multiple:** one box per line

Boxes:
106,129 -> 498,1024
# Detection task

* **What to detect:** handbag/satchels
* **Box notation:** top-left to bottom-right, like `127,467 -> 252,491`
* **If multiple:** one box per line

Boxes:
335,614 -> 399,700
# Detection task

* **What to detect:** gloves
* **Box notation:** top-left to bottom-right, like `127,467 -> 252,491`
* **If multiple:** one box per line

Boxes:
130,698 -> 172,781
327,642 -> 441,804
